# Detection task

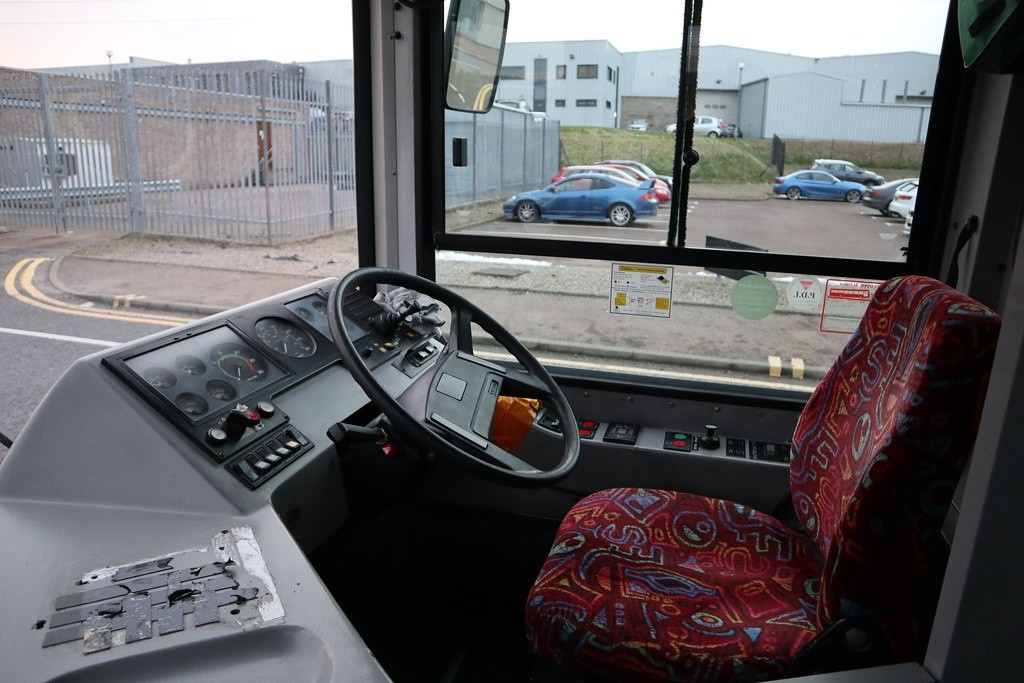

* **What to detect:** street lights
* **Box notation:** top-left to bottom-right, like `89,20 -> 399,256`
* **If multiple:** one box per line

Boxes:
734,63 -> 744,139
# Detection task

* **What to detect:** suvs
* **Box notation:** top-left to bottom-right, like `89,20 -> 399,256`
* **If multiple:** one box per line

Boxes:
495,99 -> 534,119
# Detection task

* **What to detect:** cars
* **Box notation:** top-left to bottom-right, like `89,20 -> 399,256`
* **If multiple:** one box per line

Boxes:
626,119 -> 650,132
772,170 -> 866,203
862,178 -> 919,236
551,160 -> 673,205
810,160 -> 886,189
503,173 -> 659,227
664,116 -> 742,139
532,112 -> 550,122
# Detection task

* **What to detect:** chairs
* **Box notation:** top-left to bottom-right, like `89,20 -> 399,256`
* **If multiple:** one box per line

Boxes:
519,270 -> 1003,683
